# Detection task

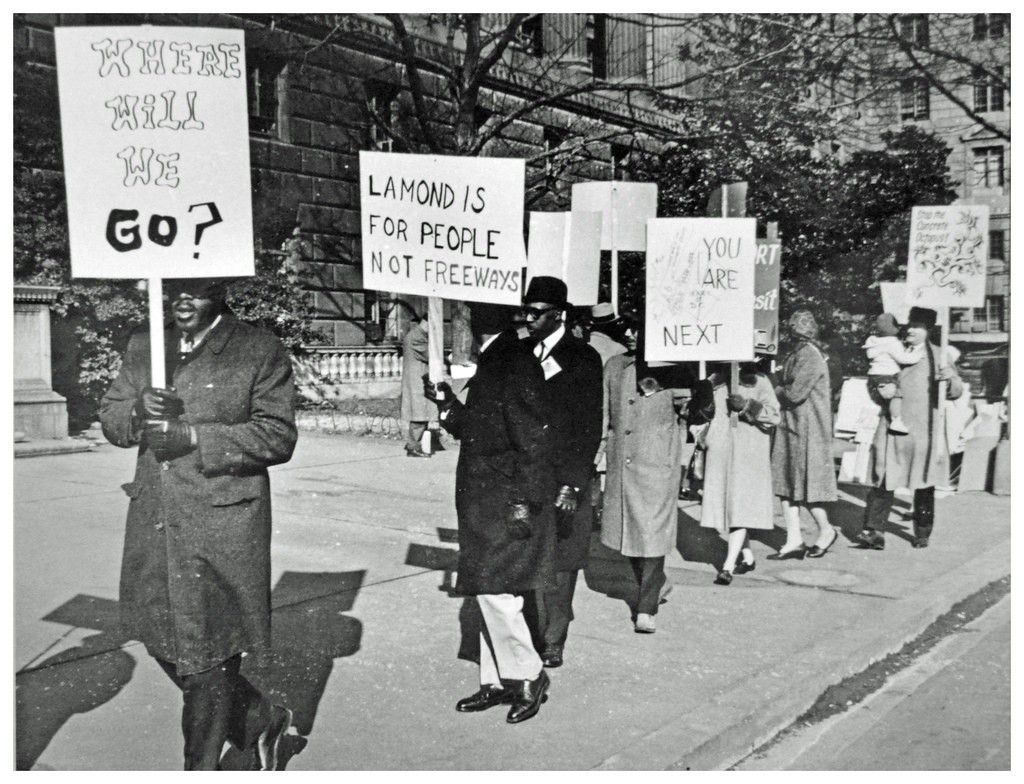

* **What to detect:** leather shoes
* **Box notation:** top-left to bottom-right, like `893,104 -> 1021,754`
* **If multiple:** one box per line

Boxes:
507,670 -> 550,723
406,448 -> 426,457
456,685 -> 516,712
249,704 -> 291,771
540,644 -> 563,667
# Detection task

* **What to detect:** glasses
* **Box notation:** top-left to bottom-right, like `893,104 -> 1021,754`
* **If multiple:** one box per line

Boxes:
520,306 -> 557,320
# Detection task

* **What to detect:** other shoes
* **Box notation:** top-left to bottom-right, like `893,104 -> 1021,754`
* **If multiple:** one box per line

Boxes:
890,420 -> 909,434
636,613 -> 656,633
717,507 -> 930,585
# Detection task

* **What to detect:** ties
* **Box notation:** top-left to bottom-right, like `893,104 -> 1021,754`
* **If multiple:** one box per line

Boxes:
185,335 -> 195,353
538,342 -> 546,359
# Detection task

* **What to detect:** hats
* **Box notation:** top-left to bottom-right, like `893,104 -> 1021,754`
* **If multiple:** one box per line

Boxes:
589,302 -> 620,325
789,310 -> 818,342
522,275 -> 574,311
876,313 -> 901,337
908,306 -> 937,329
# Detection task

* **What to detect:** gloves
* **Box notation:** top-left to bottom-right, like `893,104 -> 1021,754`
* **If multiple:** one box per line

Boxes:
728,393 -> 745,412
134,384 -> 185,421
422,374 -> 456,411
143,422 -> 192,463
505,498 -> 532,540
553,485 -> 578,514
692,380 -> 713,404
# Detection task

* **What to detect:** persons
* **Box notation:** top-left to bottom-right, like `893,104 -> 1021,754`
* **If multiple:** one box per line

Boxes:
421,277 -> 604,723
401,311 -> 432,458
690,308 -> 838,585
587,302 -> 716,634
100,278 -> 298,771
852,307 -> 964,550
